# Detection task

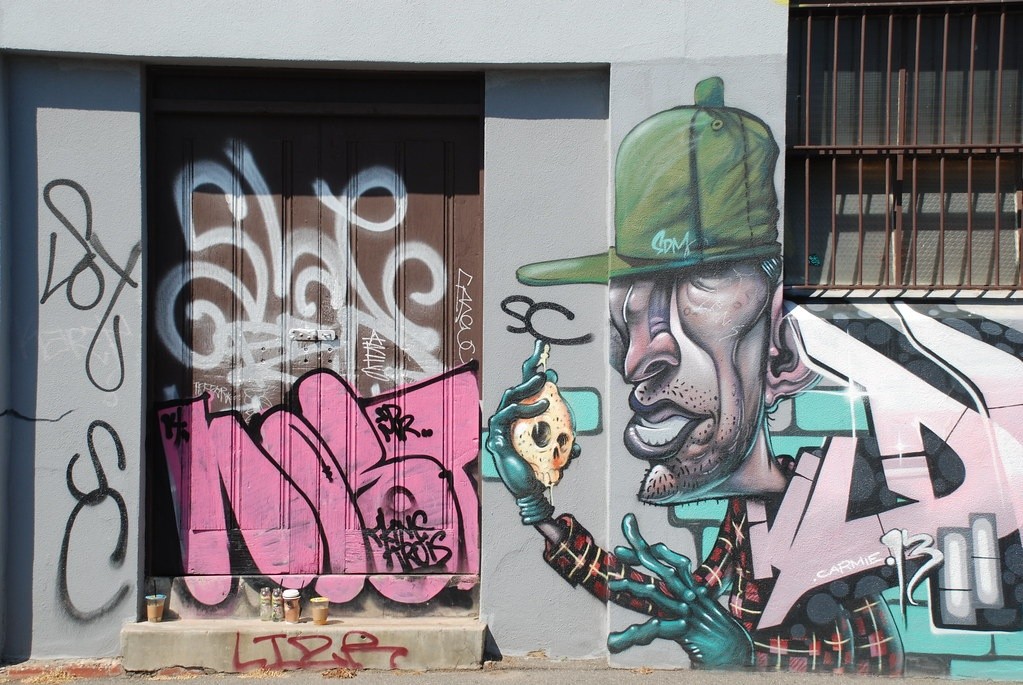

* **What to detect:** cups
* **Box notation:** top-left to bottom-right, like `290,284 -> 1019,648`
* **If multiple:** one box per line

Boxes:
310,597 -> 330,626
145,594 -> 166,622
281,596 -> 301,624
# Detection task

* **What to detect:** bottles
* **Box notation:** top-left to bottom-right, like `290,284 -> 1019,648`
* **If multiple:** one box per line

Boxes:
271,589 -> 284,622
259,587 -> 272,621
